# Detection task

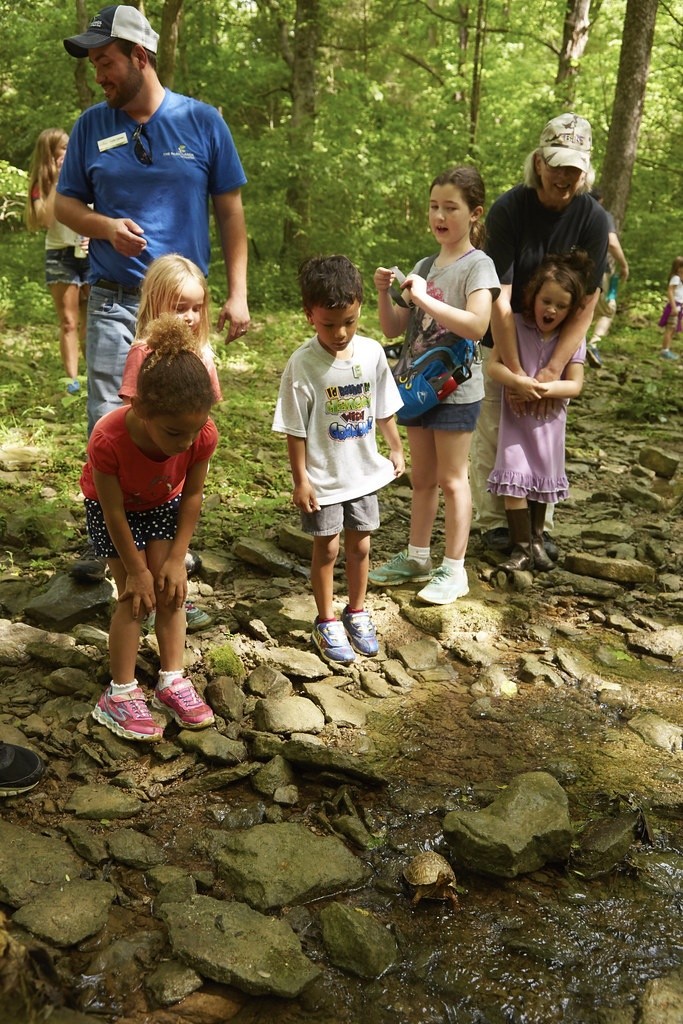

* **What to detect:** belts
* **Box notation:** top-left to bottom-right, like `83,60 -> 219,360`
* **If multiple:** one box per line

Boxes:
94,279 -> 138,296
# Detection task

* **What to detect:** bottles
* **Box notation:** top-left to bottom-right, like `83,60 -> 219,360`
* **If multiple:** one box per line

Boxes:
74,233 -> 87,258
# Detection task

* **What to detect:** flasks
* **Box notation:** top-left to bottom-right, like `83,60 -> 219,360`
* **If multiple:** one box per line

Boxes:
428,365 -> 473,400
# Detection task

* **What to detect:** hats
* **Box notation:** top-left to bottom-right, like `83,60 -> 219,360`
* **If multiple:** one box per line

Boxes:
63,5 -> 160,58
541,113 -> 593,173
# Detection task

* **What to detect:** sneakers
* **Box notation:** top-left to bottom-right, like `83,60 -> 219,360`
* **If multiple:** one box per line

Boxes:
416,565 -> 470,604
310,615 -> 356,664
90,688 -> 163,743
152,676 -> 215,729
185,603 -> 213,633
0,740 -> 46,797
368,548 -> 433,586
340,604 -> 379,656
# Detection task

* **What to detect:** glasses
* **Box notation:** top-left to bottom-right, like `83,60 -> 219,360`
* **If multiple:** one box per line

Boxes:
131,123 -> 153,166
543,156 -> 580,178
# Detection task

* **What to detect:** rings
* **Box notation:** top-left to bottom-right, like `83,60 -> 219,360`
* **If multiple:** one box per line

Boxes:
241,329 -> 246,332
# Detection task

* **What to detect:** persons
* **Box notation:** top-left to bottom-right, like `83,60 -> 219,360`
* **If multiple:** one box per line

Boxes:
488,252 -> 593,570
25,126 -> 95,391
117,254 -> 223,629
269,253 -> 409,665
657,256 -> 683,362
78,313 -> 219,743
64,6 -> 251,578
588,185 -> 629,369
467,115 -> 611,565
373,168 -> 504,604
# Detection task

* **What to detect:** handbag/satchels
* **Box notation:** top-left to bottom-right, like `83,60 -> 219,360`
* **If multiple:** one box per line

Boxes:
392,332 -> 474,421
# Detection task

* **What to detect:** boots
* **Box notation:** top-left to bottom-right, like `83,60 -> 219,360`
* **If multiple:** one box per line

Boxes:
496,508 -> 535,572
527,499 -> 556,570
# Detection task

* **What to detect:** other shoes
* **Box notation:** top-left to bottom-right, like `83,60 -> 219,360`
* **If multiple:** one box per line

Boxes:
184,553 -> 195,574
659,352 -> 678,362
542,532 -> 558,562
586,343 -> 602,369
68,379 -> 80,394
481,527 -> 512,551
